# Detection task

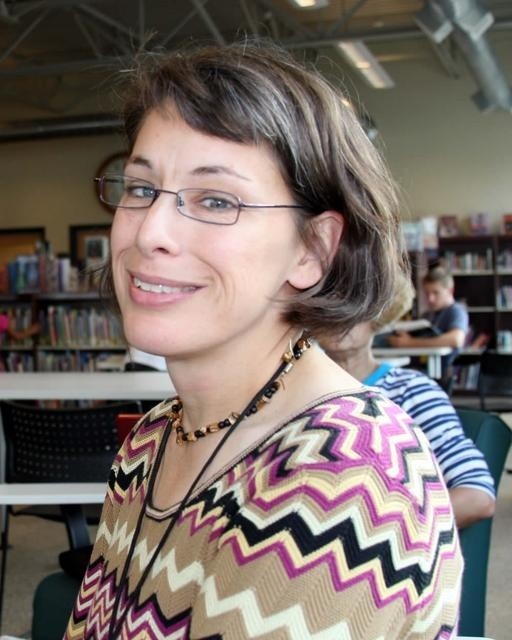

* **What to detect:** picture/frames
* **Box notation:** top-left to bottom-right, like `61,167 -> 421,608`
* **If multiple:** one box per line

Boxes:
66,223 -> 116,293
0,226 -> 47,295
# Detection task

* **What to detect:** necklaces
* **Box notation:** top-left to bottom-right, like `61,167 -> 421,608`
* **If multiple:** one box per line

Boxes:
108,333 -> 306,640
169,332 -> 315,447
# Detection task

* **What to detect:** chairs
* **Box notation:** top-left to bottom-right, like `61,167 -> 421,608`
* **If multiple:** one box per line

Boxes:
0,398 -> 127,557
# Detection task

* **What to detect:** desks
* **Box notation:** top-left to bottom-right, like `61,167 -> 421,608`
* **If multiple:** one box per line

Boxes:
1,368 -> 184,404
1,478 -> 124,511
368,345 -> 456,379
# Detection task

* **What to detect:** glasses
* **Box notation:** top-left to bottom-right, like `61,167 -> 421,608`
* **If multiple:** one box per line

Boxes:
94,172 -> 306,226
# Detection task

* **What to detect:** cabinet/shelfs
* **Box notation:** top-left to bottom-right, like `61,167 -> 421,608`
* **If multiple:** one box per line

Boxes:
1,255 -> 149,417
381,230 -> 512,409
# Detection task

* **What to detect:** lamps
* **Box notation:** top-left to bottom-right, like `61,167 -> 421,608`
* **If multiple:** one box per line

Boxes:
290,0 -> 329,12
334,0 -> 396,91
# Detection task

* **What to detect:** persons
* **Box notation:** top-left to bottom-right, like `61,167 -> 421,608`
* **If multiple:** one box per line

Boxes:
61,38 -> 466,640
30,266 -> 495,638
384,268 -> 468,378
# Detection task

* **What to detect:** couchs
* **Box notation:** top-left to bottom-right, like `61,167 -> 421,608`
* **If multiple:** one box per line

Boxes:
427,407 -> 511,638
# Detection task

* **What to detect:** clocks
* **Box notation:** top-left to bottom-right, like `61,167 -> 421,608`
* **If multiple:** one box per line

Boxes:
95,151 -> 136,217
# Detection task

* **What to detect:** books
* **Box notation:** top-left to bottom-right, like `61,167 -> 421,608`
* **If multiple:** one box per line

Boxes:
424,237 -> 512,392
1,237 -> 127,406
